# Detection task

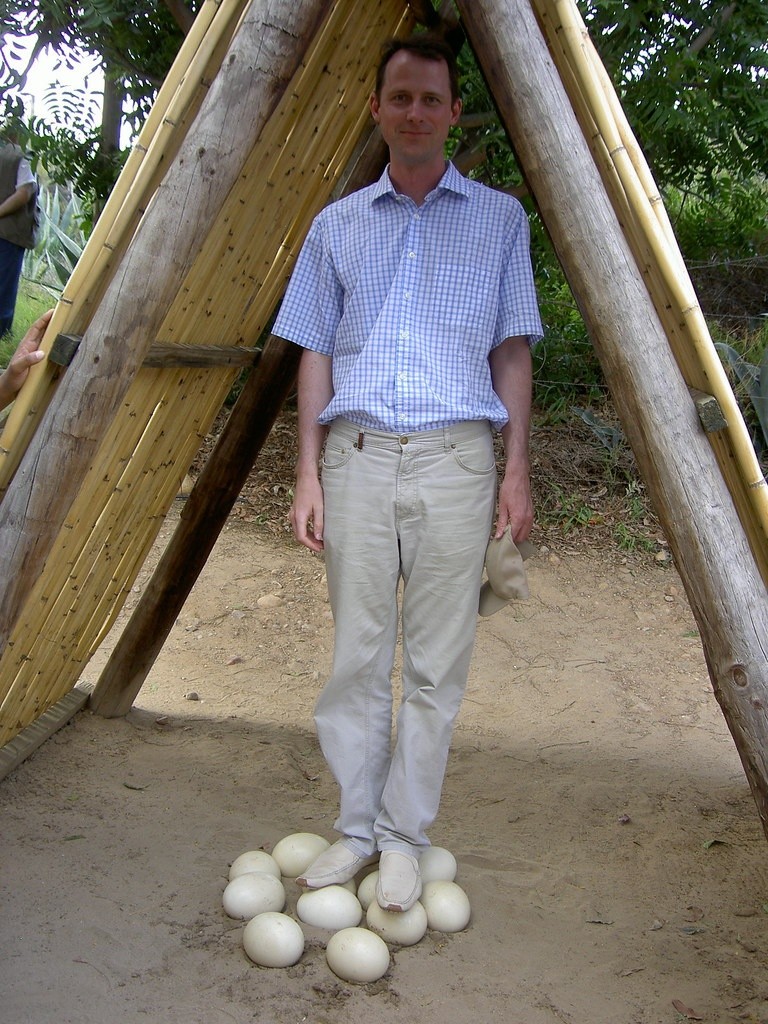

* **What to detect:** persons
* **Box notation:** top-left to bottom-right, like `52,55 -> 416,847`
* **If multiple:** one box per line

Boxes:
272,42 -> 544,914
0,132 -> 56,410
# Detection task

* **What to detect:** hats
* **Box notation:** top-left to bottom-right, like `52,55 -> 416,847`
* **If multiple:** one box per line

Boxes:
478,523 -> 537,617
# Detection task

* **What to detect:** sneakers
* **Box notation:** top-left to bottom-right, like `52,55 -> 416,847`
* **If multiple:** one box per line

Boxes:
376,849 -> 422,912
295,838 -> 381,888
